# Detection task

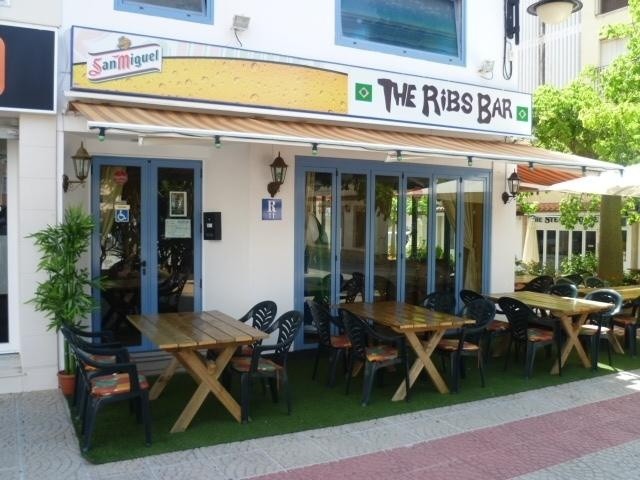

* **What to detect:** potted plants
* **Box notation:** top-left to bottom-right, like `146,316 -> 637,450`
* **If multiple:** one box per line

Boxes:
28,207 -> 108,394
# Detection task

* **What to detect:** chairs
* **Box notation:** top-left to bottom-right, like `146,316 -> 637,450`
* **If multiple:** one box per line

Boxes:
336,307 -> 412,406
303,271 -> 454,308
458,269 -> 640,382
206,301 -> 278,397
62,328 -> 152,452
100,254 -> 189,338
406,291 -> 457,373
61,322 -> 136,422
222,310 -> 302,426
431,296 -> 496,394
304,299 -> 376,388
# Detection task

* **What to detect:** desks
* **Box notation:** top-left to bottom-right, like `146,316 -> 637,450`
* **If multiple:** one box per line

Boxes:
340,300 -> 477,402
125,311 -> 270,434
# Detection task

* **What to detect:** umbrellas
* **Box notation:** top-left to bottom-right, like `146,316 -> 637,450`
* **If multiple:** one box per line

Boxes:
522,215 -> 540,272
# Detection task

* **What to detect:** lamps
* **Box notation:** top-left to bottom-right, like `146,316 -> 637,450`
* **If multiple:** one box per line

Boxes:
92,125 -> 626,180
504,0 -> 584,47
266,148 -> 288,196
500,165 -> 520,203
61,138 -> 91,194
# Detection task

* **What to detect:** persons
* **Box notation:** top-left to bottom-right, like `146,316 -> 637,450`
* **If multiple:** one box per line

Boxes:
104,253 -> 141,279
309,212 -> 329,264
